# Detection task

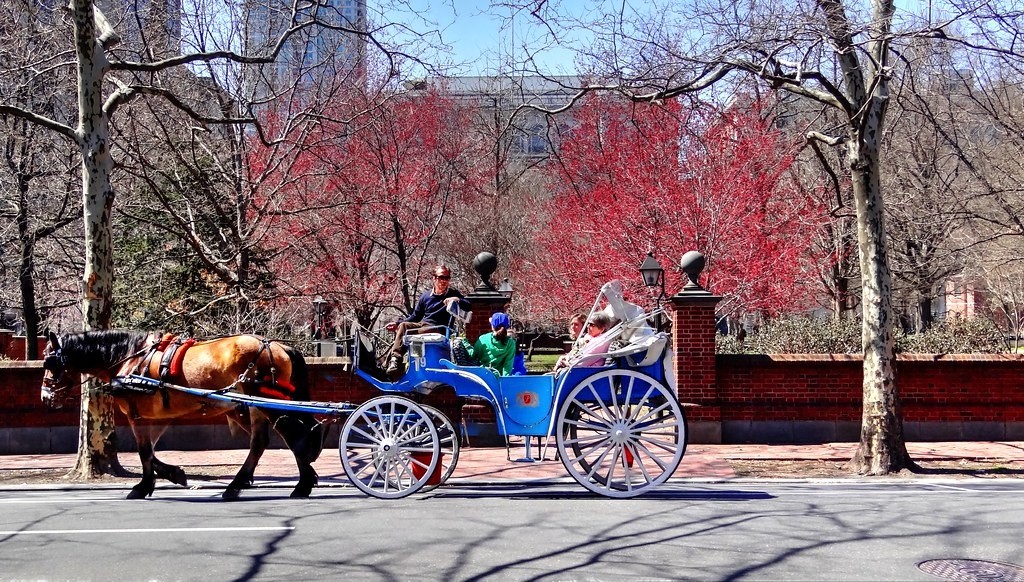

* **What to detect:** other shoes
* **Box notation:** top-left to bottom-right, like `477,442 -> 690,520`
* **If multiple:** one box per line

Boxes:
385,358 -> 401,374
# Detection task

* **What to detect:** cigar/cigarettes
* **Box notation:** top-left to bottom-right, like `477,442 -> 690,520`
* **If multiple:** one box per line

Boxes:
383,327 -> 386,329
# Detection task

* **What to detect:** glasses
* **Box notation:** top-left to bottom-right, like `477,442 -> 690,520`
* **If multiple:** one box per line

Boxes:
434,275 -> 451,280
587,322 -> 597,326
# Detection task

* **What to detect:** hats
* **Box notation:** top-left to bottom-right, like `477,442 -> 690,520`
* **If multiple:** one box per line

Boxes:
489,312 -> 509,329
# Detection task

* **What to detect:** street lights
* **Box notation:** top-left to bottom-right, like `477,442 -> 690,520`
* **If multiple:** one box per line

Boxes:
638,251 -> 664,424
312,296 -> 328,358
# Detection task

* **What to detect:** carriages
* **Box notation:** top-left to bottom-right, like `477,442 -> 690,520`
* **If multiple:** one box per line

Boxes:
40,280 -> 688,499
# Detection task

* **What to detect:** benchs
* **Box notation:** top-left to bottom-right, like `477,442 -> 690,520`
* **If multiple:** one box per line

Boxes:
555,340 -> 622,379
447,334 -> 499,376
401,299 -> 470,344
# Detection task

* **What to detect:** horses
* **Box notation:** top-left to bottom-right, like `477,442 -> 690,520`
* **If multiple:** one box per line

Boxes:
40,329 -> 319,502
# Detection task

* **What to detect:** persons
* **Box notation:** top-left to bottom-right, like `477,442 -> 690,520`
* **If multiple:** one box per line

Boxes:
542,311 -> 611,375
457,313 -> 526,377
385,264 -> 472,374
736,323 -> 745,348
